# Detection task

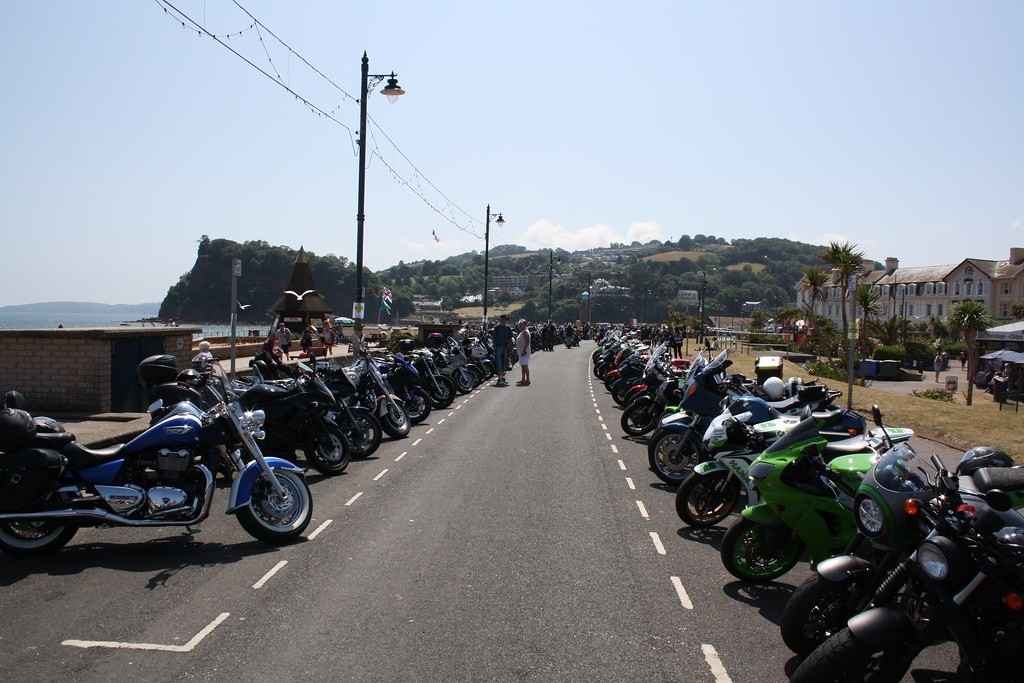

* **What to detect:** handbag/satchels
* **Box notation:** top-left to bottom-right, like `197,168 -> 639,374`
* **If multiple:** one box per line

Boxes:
288,341 -> 291,347
320,337 -> 325,343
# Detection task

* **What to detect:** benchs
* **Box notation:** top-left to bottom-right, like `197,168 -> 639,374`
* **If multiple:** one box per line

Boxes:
337,335 -> 352,344
290,347 -> 328,360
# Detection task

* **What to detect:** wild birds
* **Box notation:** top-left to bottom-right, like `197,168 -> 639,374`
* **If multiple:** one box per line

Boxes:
237,301 -> 252,310
432,229 -> 442,243
283,289 -> 316,300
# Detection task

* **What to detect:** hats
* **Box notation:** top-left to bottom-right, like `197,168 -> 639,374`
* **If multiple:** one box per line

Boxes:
498,315 -> 509,320
307,321 -> 312,325
280,323 -> 284,326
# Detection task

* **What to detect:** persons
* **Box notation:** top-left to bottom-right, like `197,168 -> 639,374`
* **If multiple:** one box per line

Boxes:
984,362 -> 1024,393
167,319 -> 176,327
565,323 -> 590,347
263,322 -> 293,379
381,326 -> 394,341
322,321 -> 343,355
960,351 -> 967,370
300,324 -> 314,353
704,337 -> 720,351
597,324 -> 605,342
192,340 -> 213,365
488,321 -> 495,335
934,356 -> 943,382
539,321 -> 557,352
838,344 -> 845,358
633,323 -> 684,359
491,315 -> 531,386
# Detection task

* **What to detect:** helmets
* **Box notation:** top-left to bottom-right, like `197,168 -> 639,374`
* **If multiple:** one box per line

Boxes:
177,369 -> 202,388
763,376 -> 785,401
990,526 -> 1024,573
0,408 -> 38,447
956,447 -> 1014,478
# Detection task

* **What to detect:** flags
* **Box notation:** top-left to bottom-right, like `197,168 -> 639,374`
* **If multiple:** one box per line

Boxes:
380,287 -> 393,316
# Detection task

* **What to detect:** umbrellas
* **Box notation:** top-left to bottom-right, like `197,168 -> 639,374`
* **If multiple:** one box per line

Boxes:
979,348 -> 1024,363
334,317 -> 355,334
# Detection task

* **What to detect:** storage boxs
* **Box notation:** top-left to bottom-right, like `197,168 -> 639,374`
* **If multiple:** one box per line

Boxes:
400,339 -> 414,352
755,356 -> 783,385
0,446 -> 63,511
958,446 -> 1014,476
138,354 -> 179,385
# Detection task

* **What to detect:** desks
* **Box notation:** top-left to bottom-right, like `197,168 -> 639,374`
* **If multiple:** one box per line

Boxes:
370,333 -> 381,343
348,342 -> 368,353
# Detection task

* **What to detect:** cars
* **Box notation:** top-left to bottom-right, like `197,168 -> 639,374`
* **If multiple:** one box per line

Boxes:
764,327 -> 775,333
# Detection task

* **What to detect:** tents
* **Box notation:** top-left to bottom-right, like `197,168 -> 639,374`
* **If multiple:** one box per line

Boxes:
976,321 -> 1024,341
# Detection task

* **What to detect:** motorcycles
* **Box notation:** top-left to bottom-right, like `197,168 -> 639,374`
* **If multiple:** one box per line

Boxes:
488,326 -> 592,370
789,453 -> 1024,683
138,340 -> 351,477
231,332 -> 495,459
0,341 -> 313,557
781,404 -> 1024,656
720,403 -> 909,585
593,328 -> 914,528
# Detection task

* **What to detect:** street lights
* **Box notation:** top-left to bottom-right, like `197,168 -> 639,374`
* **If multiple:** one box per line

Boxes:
645,287 -> 652,322
353,50 -> 405,363
483,204 -> 506,331
696,307 -> 703,344
548,251 -> 561,320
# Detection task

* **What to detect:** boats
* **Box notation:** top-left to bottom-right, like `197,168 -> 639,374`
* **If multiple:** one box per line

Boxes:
120,323 -> 131,326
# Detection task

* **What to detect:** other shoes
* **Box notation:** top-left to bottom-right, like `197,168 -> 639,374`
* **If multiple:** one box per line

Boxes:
496,382 -> 509,387
517,380 -> 530,384
